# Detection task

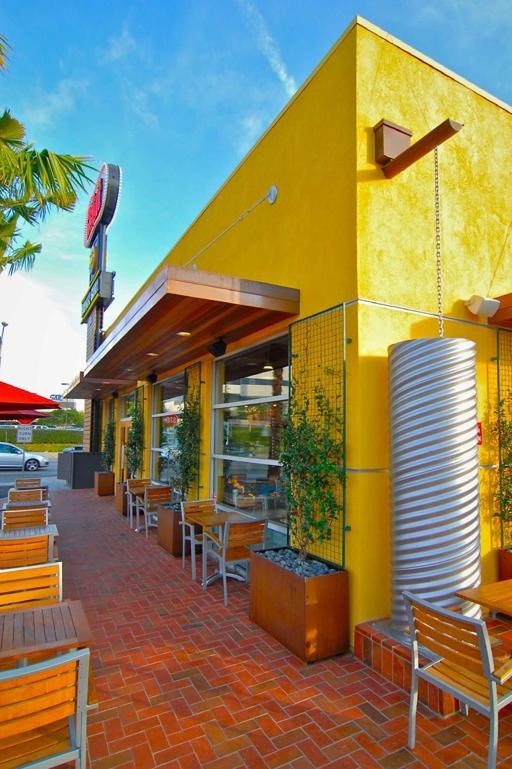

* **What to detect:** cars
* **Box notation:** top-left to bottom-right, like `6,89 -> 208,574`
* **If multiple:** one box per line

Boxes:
157,430 -> 255,467
62,444 -> 84,452
0,441 -> 49,471
0,423 -> 84,431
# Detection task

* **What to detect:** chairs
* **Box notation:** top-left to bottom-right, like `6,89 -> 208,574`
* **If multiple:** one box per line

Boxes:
1,478 -> 93,768
403,591 -> 512,769
125,479 -> 269,606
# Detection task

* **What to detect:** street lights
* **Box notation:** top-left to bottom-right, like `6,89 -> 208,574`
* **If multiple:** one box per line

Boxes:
61,381 -> 69,426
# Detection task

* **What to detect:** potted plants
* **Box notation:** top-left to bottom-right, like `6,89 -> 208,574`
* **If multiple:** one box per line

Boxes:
94,401 -> 148,516
157,385 -> 207,558
250,359 -> 347,663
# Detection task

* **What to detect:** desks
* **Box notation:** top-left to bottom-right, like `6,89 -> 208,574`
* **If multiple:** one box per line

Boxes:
454,578 -> 512,617
